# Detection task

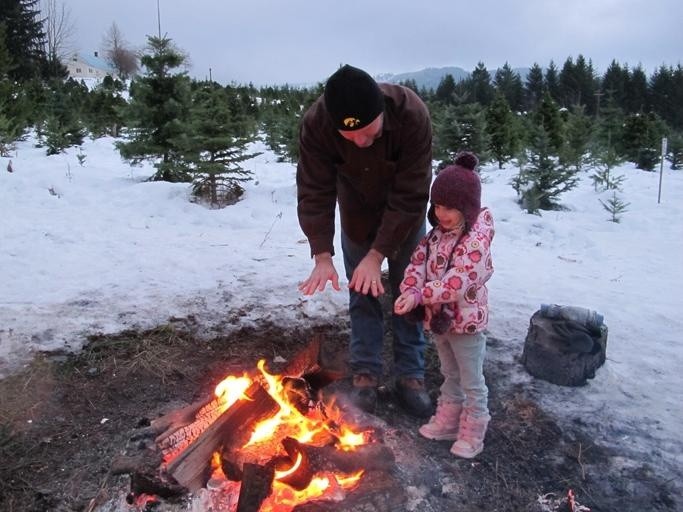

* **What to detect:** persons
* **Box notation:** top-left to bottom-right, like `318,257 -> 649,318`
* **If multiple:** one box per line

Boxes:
295,64 -> 433,421
392,152 -> 497,459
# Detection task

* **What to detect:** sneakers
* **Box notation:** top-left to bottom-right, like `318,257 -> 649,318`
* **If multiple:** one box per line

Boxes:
396,377 -> 433,418
350,375 -> 378,413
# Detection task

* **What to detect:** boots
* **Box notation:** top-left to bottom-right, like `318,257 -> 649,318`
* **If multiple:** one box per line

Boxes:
450,410 -> 490,459
419,400 -> 464,442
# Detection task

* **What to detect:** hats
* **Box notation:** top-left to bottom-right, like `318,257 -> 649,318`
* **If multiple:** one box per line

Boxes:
324,64 -> 384,130
428,153 -> 481,230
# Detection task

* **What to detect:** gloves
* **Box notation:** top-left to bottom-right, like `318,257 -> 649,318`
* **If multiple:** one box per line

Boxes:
550,320 -> 602,356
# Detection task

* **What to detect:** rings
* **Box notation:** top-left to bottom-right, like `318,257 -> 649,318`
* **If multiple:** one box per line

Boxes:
370,280 -> 376,284
